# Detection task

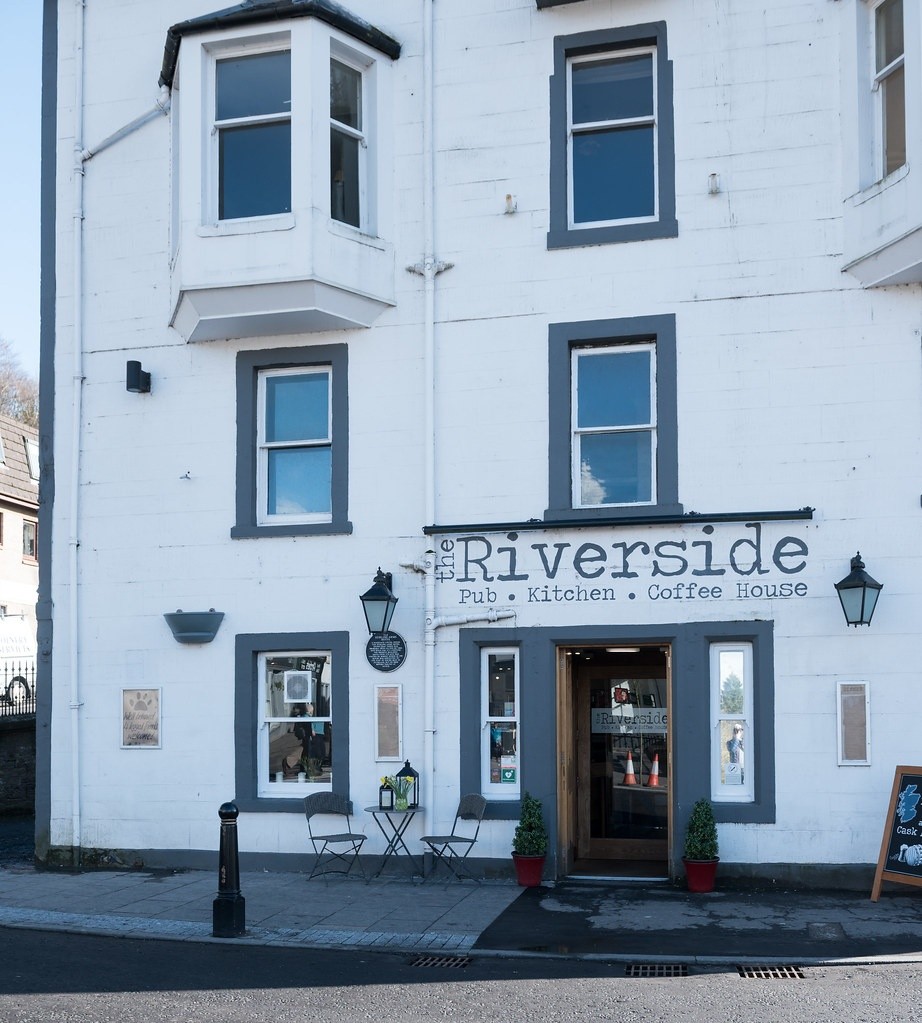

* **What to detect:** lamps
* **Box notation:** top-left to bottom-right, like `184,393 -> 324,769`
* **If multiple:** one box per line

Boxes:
834,551 -> 883,628
359,567 -> 399,635
126,361 -> 151,392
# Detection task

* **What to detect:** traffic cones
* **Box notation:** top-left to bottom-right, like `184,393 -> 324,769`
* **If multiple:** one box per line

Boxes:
618,750 -> 639,787
642,754 -> 659,787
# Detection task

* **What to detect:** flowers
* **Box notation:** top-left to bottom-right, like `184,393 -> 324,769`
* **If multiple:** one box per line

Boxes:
380,775 -> 415,799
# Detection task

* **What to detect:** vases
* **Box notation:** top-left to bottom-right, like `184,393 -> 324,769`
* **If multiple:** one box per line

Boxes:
395,797 -> 408,811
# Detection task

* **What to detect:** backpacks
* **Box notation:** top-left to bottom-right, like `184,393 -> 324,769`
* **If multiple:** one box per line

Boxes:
293,721 -> 306,739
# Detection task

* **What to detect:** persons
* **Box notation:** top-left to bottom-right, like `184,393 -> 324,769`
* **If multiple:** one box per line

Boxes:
726,723 -> 744,784
286,703 -> 325,779
491,722 -> 501,763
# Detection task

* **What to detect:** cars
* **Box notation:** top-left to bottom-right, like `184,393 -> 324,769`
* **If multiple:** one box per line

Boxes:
0,617 -> 38,705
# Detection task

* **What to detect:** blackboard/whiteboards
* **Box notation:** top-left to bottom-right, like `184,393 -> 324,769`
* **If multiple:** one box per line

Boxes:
876,764 -> 921,886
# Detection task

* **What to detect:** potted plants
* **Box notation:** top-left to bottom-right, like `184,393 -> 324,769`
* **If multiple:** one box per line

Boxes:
681,796 -> 720,893
511,790 -> 550,887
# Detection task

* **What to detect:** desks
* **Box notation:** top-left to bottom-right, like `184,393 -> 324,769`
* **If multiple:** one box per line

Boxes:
364,805 -> 425,887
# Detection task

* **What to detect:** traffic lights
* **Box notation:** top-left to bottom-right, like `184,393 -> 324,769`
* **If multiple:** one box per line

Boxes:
614,688 -> 628,703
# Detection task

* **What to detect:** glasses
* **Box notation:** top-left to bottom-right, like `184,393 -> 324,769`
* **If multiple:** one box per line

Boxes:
733,728 -> 744,734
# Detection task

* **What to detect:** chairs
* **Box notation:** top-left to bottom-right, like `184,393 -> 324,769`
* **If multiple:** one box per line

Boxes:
420,792 -> 486,892
303,791 -> 369,887
